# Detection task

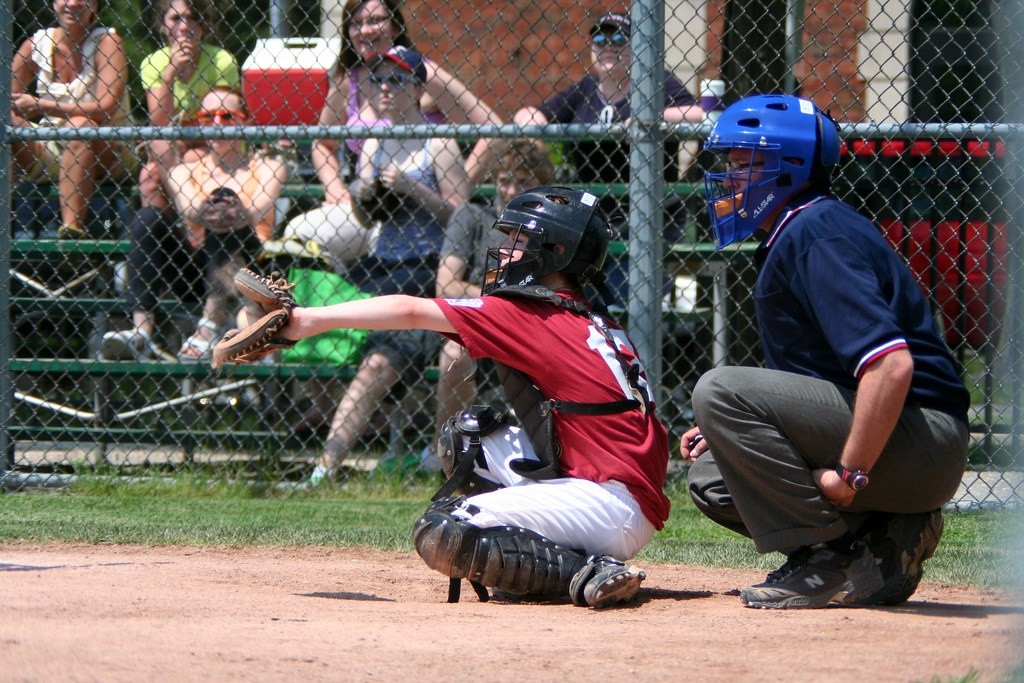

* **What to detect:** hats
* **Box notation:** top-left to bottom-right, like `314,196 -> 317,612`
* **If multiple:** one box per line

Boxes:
364,44 -> 428,83
589,12 -> 632,37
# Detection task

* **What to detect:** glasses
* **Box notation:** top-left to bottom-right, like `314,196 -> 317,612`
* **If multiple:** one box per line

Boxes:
368,72 -> 420,89
591,32 -> 628,47
194,109 -> 248,126
722,160 -> 763,173
350,16 -> 391,26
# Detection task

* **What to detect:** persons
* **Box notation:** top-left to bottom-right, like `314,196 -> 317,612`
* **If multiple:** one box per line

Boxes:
213,186 -> 670,609
101,0 -> 705,492
12,0 -> 135,241
680,95 -> 970,609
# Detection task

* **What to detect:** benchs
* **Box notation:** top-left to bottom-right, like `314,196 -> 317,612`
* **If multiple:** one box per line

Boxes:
8,137 -> 766,479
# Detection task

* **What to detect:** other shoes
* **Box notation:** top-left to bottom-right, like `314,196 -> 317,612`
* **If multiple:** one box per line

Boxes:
58,225 -> 85,241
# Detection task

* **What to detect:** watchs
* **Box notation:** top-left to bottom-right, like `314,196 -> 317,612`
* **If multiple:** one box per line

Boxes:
835,463 -> 871,491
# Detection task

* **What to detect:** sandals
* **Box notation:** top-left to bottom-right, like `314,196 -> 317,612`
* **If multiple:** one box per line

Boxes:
101,328 -> 156,359
178,309 -> 236,360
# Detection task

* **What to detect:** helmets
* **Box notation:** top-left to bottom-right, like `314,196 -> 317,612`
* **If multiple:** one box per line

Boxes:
479,186 -> 610,293
699,95 -> 842,251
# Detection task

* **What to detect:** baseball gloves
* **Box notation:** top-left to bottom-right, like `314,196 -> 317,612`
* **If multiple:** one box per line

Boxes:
212,267 -> 297,373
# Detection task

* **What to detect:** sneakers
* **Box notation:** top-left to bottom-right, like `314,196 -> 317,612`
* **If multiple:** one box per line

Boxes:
738,534 -> 882,608
868,511 -> 945,605
568,552 -> 647,608
